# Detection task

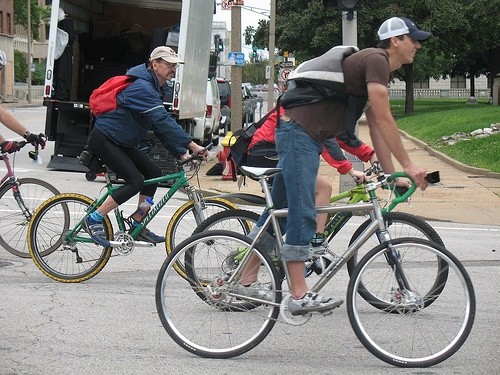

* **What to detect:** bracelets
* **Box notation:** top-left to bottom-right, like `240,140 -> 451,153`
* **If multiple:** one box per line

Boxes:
23,131 -> 30,138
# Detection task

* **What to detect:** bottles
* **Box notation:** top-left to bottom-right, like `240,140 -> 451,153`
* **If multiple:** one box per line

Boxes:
131,198 -> 154,223
312,253 -> 333,276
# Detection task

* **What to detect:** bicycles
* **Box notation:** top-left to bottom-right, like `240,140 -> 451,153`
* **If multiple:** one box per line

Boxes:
27,150 -> 253,287
184,162 -> 448,314
154,166 -> 476,368
0,132 -> 70,258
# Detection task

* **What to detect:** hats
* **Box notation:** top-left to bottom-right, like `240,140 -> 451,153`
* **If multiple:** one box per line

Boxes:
377,16 -> 431,41
149,46 -> 185,64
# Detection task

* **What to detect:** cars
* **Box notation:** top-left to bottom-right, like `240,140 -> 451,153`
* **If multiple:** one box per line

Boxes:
217,79 -> 232,136
204,77 -> 221,151
242,85 -> 257,124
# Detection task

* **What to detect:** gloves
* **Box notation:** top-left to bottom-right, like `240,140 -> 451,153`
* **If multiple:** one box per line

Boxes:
0,140 -> 21,154
23,131 -> 46,149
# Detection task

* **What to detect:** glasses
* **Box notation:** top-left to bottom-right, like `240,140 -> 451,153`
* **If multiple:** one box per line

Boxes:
157,61 -> 179,68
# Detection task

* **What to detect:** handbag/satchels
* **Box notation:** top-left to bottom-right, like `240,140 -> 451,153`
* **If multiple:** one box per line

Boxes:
229,121 -> 258,166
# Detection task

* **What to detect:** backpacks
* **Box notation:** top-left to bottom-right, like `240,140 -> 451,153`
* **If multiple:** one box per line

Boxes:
89,74 -> 149,117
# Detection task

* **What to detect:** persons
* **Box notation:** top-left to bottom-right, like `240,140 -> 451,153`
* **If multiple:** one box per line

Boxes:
236,101 -> 382,264
83,46 -> 209,246
0,107 -> 45,154
236,16 -> 429,316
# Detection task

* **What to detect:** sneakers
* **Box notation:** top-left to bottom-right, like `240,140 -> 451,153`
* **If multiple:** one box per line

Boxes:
127,224 -> 165,243
309,242 -> 338,264
81,214 -> 110,247
234,280 -> 274,301
288,290 -> 344,316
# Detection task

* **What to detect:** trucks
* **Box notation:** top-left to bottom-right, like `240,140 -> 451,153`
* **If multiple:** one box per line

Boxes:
43,1 -> 224,172
